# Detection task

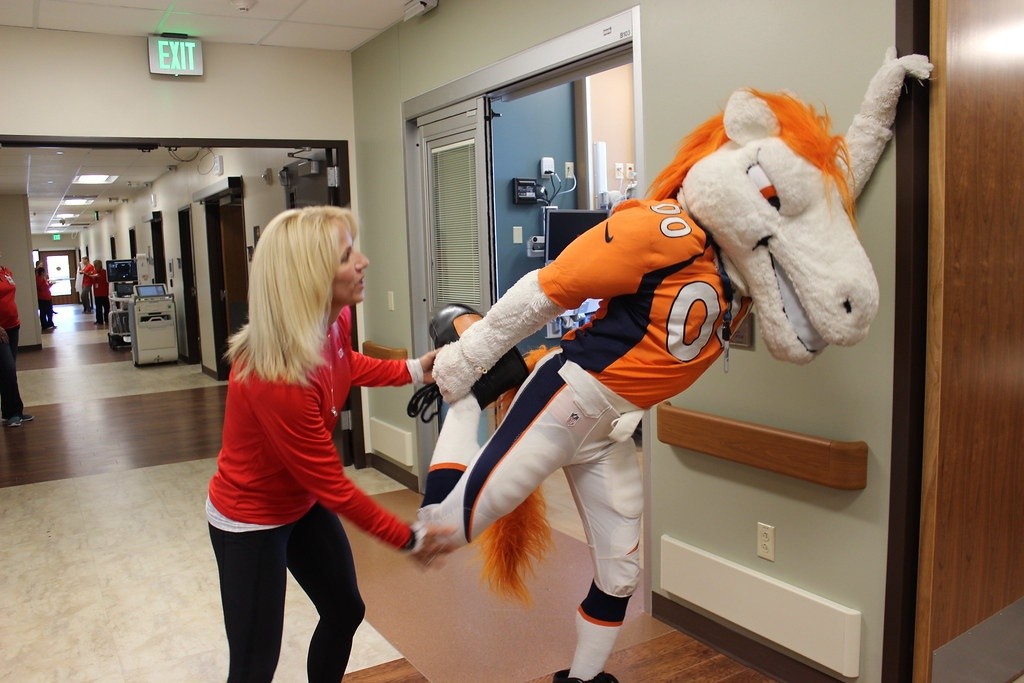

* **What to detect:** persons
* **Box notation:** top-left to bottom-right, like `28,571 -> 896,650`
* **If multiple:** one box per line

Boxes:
0,260 -> 36,428
33,255 -> 114,332
203,203 -> 464,683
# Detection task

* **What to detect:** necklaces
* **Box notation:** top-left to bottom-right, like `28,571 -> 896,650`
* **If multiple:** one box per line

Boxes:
315,333 -> 339,417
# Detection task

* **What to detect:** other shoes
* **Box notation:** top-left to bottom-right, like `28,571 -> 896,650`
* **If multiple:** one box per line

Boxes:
427,300 -> 529,410
553,669 -> 620,683
104,319 -> 108,322
42,325 -> 57,330
94,321 -> 103,325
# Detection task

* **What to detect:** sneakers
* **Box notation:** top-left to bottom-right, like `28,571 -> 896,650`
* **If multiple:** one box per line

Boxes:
1,413 -> 35,426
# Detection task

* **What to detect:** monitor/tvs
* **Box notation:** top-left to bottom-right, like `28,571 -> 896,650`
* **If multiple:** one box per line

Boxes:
117,283 -> 134,297
133,283 -> 167,296
544,209 -> 611,265
105,259 -> 138,283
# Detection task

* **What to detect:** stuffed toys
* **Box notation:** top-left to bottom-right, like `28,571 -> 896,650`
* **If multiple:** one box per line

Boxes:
409,42 -> 935,683
